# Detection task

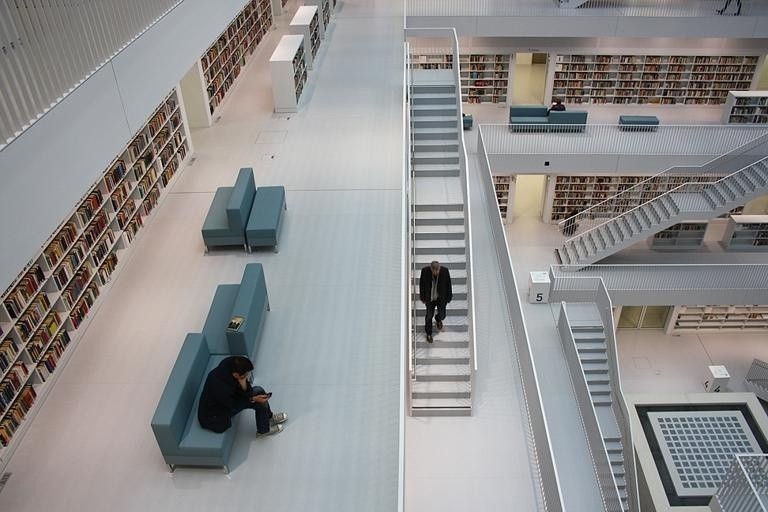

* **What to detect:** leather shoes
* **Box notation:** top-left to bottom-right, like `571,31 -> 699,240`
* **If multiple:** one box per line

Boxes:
426,334 -> 433,343
435,315 -> 442,329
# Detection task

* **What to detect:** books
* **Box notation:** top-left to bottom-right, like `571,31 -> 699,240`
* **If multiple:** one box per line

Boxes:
226,315 -> 245,332
492,176 -> 768,245
423,56 -> 453,70
468,55 -> 504,104
292,0 -> 337,104
553,56 -> 760,105
106,98 -> 187,247
201,0 -> 288,116
0,188 -> 118,449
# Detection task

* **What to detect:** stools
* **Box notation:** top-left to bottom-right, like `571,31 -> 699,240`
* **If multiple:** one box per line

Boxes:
247,188 -> 287,253
620,114 -> 665,133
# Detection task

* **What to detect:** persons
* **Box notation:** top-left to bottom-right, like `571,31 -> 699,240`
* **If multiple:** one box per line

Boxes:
420,261 -> 452,344
716,0 -> 741,16
198,356 -> 288,438
547,99 -> 566,116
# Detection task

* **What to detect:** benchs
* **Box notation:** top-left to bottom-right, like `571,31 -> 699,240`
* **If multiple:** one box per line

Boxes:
509,104 -> 587,133
201,264 -> 274,359
201,169 -> 256,253
151,329 -> 251,482
463,115 -> 473,128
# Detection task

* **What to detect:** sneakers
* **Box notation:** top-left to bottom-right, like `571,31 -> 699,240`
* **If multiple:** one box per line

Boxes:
256,412 -> 288,438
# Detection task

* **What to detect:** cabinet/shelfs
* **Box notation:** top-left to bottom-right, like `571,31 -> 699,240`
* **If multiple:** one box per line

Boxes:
665,306 -> 768,339
724,91 -> 768,127
0,83 -> 194,479
538,176 -> 768,251
550,55 -> 758,107
181,0 -> 337,126
411,55 -> 508,105
495,175 -> 516,223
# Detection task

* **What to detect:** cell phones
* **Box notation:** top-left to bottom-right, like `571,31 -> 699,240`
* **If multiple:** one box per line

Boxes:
266,392 -> 273,398
